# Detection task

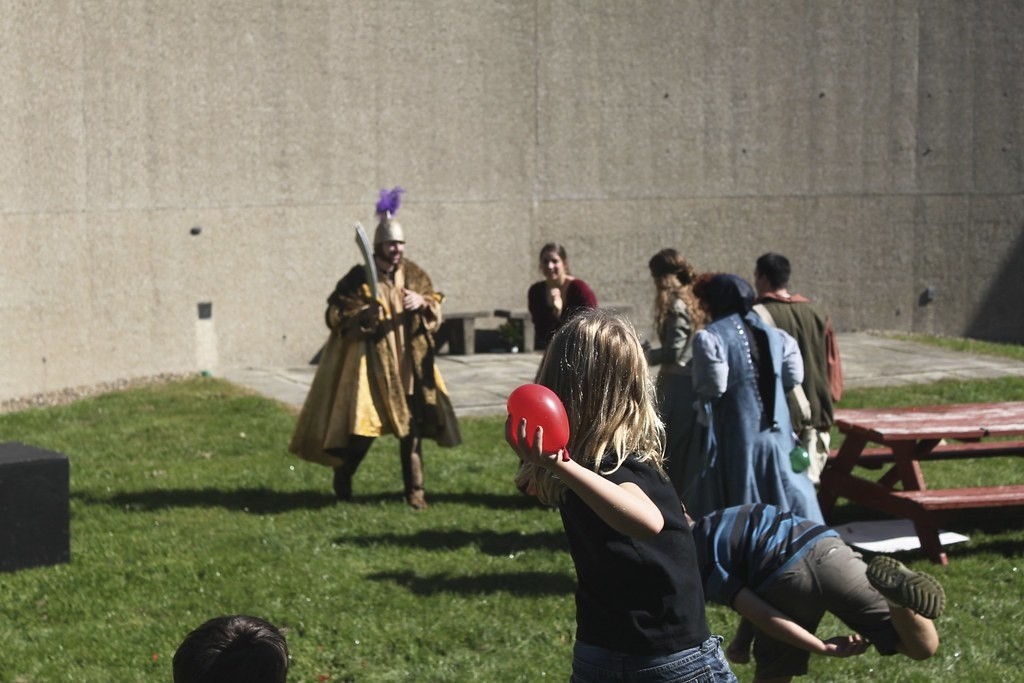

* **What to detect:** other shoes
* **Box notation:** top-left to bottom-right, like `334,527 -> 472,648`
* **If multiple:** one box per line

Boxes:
865,554 -> 947,618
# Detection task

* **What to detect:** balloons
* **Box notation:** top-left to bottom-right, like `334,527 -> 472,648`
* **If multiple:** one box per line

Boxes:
507,384 -> 571,462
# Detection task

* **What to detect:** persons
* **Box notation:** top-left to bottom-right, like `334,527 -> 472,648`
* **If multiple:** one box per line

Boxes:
641,247 -> 843,525
290,187 -> 463,509
172,615 -> 289,683
681,503 -> 947,683
504,306 -> 740,683
528,243 -> 598,351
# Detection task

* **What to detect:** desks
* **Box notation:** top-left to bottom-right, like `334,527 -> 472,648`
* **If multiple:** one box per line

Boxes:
817,400 -> 1024,566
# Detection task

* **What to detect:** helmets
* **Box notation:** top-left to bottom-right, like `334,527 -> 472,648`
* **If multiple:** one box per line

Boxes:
372,184 -> 407,245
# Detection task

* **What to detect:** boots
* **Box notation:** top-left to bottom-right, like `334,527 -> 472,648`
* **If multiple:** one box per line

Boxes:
400,437 -> 430,508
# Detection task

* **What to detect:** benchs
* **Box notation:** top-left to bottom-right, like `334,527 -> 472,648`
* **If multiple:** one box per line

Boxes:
880,484 -> 1024,535
827,441 -> 1024,470
443,311 -> 489,355
494,304 -> 635,353
